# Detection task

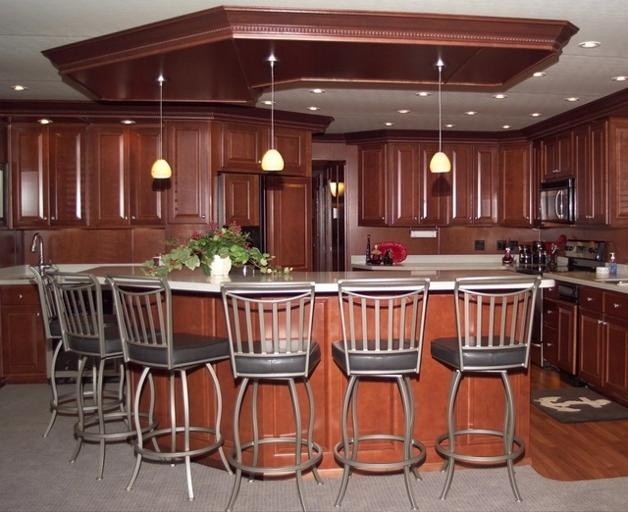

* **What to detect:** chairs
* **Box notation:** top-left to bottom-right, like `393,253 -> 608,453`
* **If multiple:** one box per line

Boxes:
105,273 -> 234,501
219,280 -> 329,511
28,263 -> 133,440
41,269 -> 170,482
328,273 -> 433,511
436,271 -> 543,508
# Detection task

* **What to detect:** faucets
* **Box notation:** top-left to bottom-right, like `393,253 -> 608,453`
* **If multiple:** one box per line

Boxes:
30,233 -> 45,264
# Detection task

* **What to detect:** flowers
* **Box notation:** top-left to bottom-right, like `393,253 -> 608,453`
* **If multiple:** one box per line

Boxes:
141,216 -> 277,283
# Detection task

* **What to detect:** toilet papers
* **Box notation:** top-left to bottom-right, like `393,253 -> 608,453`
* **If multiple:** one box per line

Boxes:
410,230 -> 437,238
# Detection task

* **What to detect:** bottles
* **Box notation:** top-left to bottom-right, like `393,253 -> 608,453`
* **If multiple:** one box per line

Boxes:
383,248 -> 394,265
532,240 -> 546,264
365,234 -> 372,262
518,244 -> 531,264
502,247 -> 513,264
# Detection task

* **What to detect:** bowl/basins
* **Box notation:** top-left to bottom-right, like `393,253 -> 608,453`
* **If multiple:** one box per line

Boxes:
556,266 -> 568,272
555,256 -> 568,266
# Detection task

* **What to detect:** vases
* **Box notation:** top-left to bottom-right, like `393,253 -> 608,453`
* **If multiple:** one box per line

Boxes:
202,255 -> 234,276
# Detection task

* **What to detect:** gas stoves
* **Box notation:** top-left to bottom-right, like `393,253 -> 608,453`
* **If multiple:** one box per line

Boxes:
516,264 -> 592,276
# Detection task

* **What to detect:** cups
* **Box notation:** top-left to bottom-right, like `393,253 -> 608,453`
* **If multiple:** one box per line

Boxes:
596,267 -> 608,274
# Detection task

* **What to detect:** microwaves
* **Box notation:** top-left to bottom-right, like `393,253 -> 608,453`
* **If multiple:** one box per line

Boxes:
538,176 -> 575,226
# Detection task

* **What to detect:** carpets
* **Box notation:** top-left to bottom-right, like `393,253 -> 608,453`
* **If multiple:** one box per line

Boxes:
528,377 -> 628,427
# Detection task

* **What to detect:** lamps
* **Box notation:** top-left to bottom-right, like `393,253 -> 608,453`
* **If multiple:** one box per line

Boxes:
151,75 -> 174,182
258,54 -> 289,177
427,54 -> 456,177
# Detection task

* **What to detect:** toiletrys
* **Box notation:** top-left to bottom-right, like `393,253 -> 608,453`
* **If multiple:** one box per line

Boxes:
609,252 -> 617,278
503,247 -> 515,265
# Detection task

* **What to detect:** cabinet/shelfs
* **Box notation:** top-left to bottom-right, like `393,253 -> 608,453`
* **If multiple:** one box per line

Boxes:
218,174 -> 262,228
579,290 -> 628,407
571,113 -> 627,231
539,130 -> 571,180
386,138 -> 449,229
266,178 -> 311,272
1,103 -> 77,227
214,115 -> 315,174
451,137 -> 533,228
356,141 -> 386,228
545,282 -> 578,380
1,283 -> 49,383
80,104 -> 211,233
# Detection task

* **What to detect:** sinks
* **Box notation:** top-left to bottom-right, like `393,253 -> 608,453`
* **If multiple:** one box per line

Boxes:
0,263 -> 94,285
